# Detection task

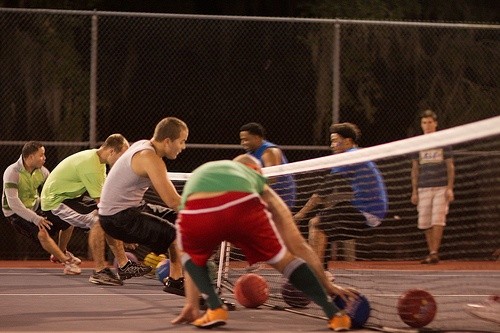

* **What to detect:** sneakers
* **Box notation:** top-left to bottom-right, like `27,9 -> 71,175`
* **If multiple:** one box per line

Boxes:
194,306 -> 228,327
64,254 -> 82,275
116,259 -> 153,279
50,248 -> 81,265
88,266 -> 124,286
327,311 -> 353,331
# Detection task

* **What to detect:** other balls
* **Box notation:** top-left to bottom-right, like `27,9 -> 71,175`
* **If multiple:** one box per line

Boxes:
333,291 -> 370,329
281,280 -> 310,308
234,274 -> 270,308
155,258 -> 170,283
113,251 -> 140,276
145,252 -> 167,275
397,290 -> 437,328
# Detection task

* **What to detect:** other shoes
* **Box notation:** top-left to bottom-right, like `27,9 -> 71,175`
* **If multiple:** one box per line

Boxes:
420,253 -> 439,264
324,270 -> 336,283
244,261 -> 261,273
162,277 -> 206,305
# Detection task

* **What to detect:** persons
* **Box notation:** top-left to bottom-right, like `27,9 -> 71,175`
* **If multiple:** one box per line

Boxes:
1,142 -> 81,275
98,117 -> 237,310
292,123 -> 388,281
40,134 -> 152,286
239,123 -> 296,272
410,110 -> 455,265
172,152 -> 359,331
